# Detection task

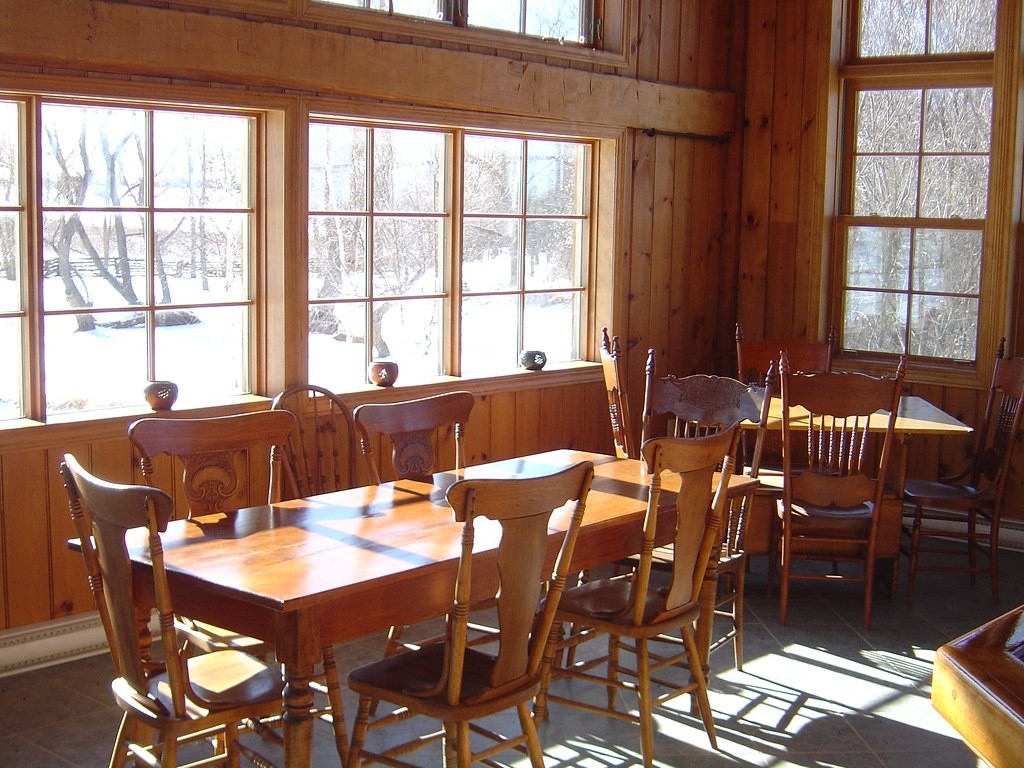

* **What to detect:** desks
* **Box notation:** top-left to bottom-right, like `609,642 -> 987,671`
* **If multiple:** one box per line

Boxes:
679,394 -> 974,598
64,444 -> 759,766
928,602 -> 1024,767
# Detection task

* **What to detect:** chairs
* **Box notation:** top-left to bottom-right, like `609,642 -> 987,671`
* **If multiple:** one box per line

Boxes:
60,336 -> 1024,767
